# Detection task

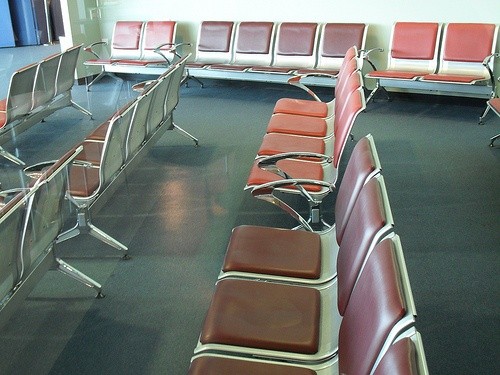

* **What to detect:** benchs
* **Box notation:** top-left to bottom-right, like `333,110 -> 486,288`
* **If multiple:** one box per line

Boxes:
364,21 -> 500,124
188,134 -> 429,375
24,53 -> 198,260
244,46 -> 366,232
486,76 -> 500,147
84,20 -> 178,92
0,43 -> 93,169
0,145 -> 106,313
170,21 -> 374,104
